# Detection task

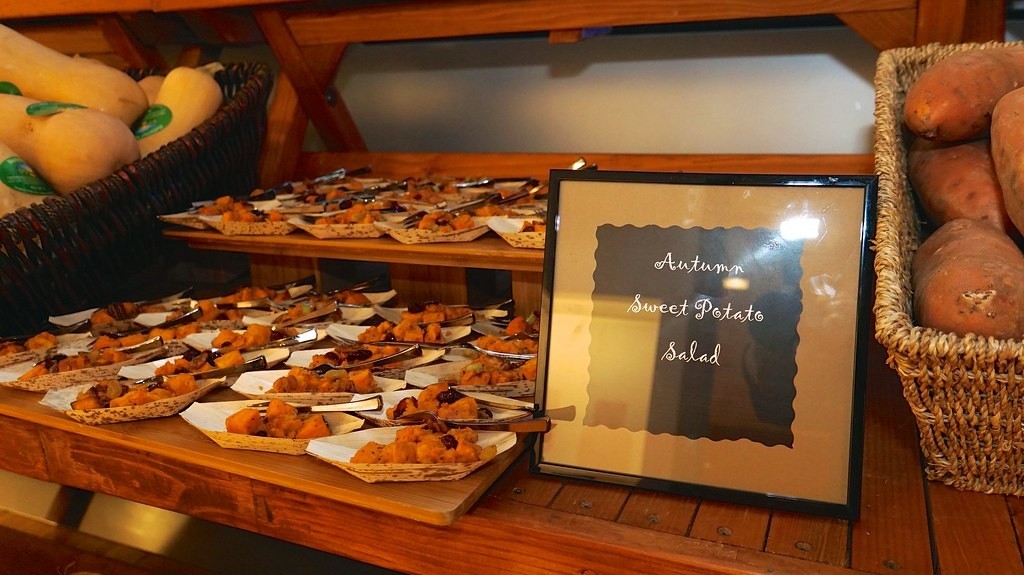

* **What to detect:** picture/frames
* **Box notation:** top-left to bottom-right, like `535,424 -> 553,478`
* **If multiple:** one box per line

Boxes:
528,169 -> 879,524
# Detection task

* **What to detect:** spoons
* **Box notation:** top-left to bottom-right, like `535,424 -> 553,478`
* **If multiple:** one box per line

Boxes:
33,335 -> 164,367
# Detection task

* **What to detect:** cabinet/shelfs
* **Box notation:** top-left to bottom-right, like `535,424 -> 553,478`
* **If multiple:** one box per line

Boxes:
0,0 -> 1024,575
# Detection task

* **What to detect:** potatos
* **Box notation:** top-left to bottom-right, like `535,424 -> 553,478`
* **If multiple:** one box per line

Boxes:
903,46 -> 1024,342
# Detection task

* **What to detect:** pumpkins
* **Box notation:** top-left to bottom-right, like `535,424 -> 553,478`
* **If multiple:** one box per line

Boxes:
0,21 -> 228,221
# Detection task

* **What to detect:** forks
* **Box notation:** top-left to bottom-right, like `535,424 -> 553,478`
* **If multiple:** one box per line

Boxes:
534,208 -> 547,219
403,193 -> 503,228
396,411 -> 551,434
188,191 -> 275,216
87,306 -> 203,351
132,355 -> 268,391
505,332 -> 539,341
311,344 -> 422,377
267,297 -> 375,312
248,395 -> 383,418
331,335 -> 469,348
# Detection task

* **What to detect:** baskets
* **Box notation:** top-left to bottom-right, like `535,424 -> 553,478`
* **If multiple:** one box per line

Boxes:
869,40 -> 1024,497
1,60 -> 275,338
